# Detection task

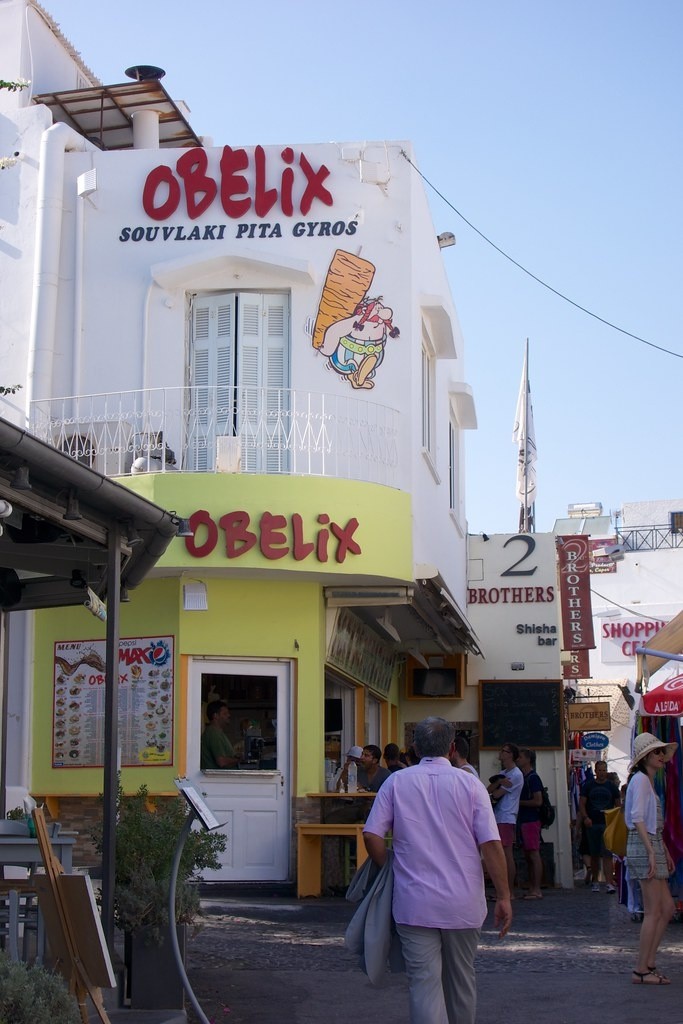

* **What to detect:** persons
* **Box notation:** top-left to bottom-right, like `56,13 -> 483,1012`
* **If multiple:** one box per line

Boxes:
363,717 -> 512,1024
331,737 -> 628,902
201,702 -> 244,769
625,732 -> 678,984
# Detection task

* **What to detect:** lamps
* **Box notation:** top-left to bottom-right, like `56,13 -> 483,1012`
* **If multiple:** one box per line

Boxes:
63,488 -> 82,520
377,606 -> 400,642
120,581 -> 129,603
409,647 -> 430,668
70,569 -> 85,587
169,510 -> 193,537
10,459 -> 31,489
437,231 -> 456,249
126,519 -> 144,547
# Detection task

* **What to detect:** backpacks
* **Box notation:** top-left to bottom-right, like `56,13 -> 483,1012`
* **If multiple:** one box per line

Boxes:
527,773 -> 555,830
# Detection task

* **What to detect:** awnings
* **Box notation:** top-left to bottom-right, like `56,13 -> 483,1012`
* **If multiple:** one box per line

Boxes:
635,610 -> 683,691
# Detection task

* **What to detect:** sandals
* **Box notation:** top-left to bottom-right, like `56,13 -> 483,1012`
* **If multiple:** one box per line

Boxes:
632,966 -> 671,984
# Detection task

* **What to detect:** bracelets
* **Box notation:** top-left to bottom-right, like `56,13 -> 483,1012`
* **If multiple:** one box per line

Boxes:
583,816 -> 588,821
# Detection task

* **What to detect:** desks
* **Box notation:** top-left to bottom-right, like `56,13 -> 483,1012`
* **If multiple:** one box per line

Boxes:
0,837 -> 76,874
307,791 -> 377,798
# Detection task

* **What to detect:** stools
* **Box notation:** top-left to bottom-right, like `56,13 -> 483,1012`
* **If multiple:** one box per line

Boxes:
296,822 -> 366,897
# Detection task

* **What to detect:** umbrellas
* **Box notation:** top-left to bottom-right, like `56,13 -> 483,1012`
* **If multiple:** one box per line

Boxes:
639,673 -> 683,717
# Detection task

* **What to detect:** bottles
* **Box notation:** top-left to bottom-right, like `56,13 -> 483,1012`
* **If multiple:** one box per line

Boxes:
325,757 -> 331,778
348,760 -> 357,794
325,738 -> 340,762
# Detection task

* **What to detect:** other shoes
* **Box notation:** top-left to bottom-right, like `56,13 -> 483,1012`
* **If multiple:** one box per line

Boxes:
592,883 -> 599,891
585,866 -> 592,885
607,884 -> 615,893
524,895 -> 544,900
485,895 -> 496,902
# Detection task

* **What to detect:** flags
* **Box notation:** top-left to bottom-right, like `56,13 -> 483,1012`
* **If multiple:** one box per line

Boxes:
513,338 -> 538,509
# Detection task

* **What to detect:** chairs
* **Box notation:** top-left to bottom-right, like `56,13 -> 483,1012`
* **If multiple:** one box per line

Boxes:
0,818 -> 62,965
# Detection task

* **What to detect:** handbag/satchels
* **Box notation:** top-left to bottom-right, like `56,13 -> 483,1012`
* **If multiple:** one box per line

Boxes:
604,784 -> 628,856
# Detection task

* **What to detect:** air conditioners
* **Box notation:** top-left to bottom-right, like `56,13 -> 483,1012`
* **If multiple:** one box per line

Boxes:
47,418 -> 137,476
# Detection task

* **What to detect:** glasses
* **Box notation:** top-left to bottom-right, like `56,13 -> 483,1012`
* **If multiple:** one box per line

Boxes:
501,749 -> 509,753
526,747 -> 532,758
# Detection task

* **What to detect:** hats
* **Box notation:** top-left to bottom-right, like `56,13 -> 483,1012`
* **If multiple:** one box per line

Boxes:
627,732 -> 679,773
342,746 -> 363,758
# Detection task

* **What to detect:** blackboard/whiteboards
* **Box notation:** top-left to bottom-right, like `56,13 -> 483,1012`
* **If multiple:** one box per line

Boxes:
477,679 -> 565,752
